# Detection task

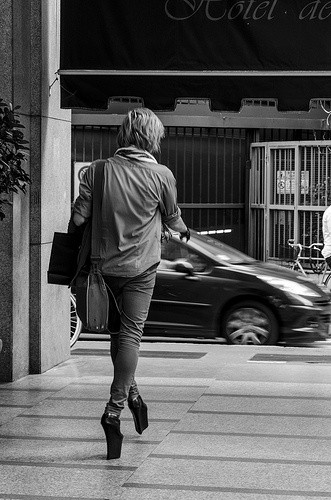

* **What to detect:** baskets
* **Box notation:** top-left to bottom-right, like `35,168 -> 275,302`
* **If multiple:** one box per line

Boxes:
278,244 -> 297,262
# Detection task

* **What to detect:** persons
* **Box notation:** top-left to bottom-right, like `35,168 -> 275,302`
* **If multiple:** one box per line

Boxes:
157,246 -> 191,271
321,206 -> 331,274
71,107 -> 190,461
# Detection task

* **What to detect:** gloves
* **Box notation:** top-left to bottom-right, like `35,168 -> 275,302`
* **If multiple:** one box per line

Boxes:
180,225 -> 190,243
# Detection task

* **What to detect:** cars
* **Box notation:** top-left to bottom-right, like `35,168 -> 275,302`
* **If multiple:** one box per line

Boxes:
74,228 -> 331,345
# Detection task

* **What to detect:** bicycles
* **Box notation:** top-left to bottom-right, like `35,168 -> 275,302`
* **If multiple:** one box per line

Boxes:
287,239 -> 331,290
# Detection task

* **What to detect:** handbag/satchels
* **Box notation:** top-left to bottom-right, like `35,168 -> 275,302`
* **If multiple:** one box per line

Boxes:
47,231 -> 82,286
76,270 -> 108,333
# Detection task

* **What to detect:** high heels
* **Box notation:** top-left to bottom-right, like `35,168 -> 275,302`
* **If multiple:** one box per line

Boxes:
129,397 -> 148,436
101,414 -> 123,460
